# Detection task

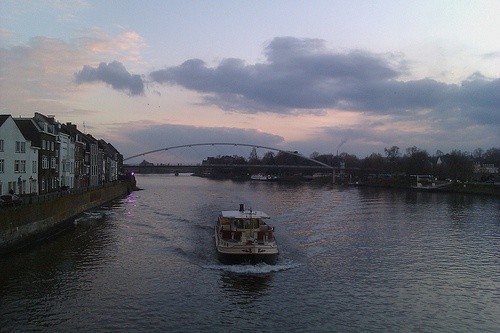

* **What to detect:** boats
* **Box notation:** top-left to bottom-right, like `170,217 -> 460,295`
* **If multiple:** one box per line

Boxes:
213,203 -> 280,265
